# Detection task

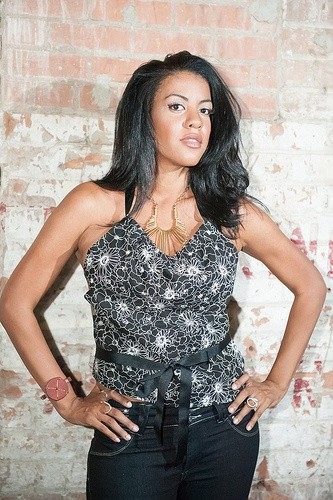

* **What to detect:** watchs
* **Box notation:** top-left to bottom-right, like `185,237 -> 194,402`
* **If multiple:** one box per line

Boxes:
41,375 -> 71,402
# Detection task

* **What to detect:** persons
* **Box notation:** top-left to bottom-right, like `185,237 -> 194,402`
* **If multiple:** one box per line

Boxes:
0,45 -> 327,500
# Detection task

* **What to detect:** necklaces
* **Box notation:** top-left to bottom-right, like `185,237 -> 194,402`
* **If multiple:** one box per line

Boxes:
138,181 -> 193,254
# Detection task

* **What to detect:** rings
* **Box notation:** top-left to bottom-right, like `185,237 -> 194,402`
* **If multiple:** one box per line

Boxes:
100,400 -> 112,414
100,389 -> 109,401
246,399 -> 257,408
